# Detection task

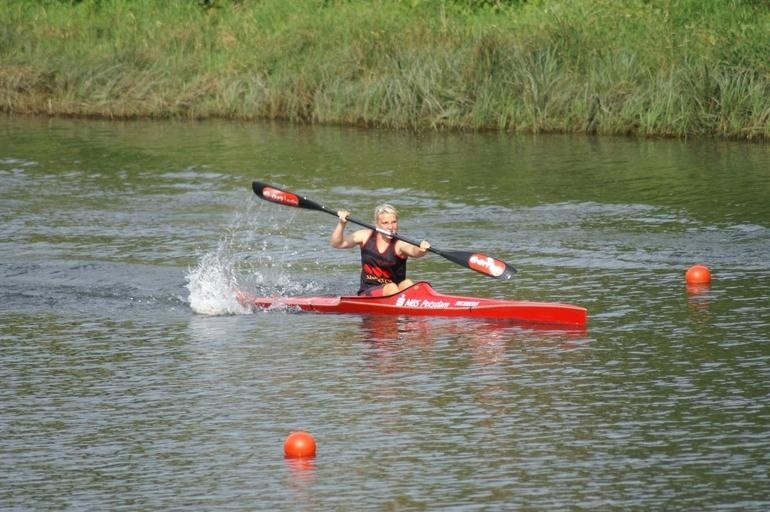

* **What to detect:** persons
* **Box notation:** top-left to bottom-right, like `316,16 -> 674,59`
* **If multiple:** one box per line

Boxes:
331,202 -> 433,298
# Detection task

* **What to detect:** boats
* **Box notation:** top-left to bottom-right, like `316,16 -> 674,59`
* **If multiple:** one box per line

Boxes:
237,281 -> 587,328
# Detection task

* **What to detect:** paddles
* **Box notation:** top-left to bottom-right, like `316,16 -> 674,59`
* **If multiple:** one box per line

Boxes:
250,180 -> 518,283
